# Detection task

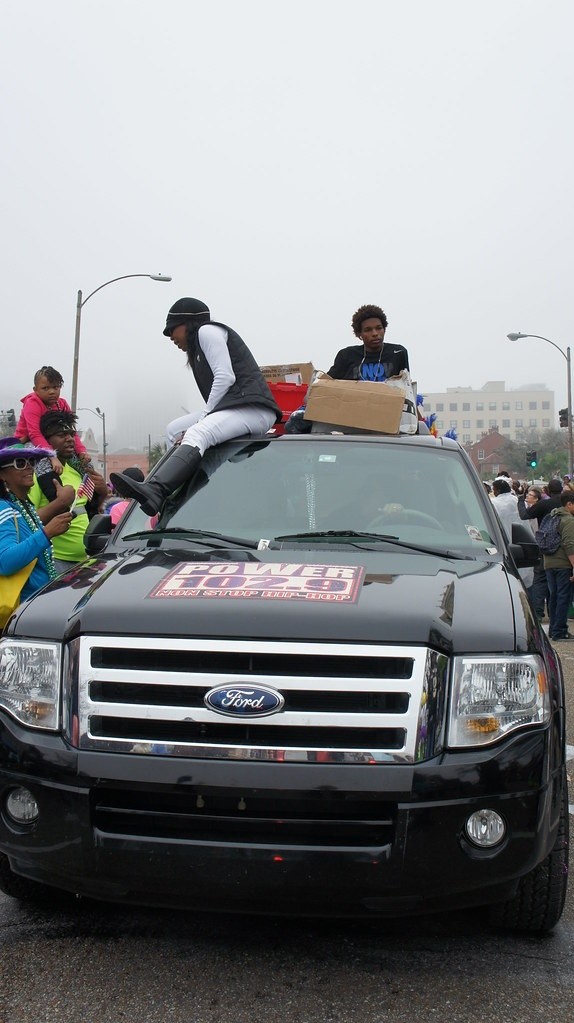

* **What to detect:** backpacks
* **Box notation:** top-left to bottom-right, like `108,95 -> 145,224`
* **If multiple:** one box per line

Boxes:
535,511 -> 563,555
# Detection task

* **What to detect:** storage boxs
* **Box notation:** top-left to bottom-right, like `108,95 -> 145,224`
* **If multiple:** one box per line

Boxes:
266,380 -> 308,423
303,368 -> 418,435
259,363 -> 315,385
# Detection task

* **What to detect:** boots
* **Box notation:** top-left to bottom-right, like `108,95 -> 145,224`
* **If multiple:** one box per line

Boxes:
110,444 -> 202,517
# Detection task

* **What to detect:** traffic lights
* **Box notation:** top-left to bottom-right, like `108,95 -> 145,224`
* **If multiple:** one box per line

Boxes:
525,451 -> 536,469
559,408 -> 568,427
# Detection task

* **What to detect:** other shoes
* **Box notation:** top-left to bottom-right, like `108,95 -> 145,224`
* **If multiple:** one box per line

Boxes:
554,632 -> 574,642
283,405 -> 313,434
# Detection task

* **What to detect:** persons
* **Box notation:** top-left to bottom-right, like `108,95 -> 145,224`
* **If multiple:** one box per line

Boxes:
12,364 -> 99,523
21,409 -> 107,578
0,437 -> 74,639
324,304 -> 411,383
40,558 -> 107,595
101,467 -> 144,532
482,471 -> 574,642
378,503 -> 409,522
110,297 -> 285,518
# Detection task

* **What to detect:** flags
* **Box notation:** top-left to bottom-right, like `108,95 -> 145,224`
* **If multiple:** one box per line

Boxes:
77,474 -> 96,502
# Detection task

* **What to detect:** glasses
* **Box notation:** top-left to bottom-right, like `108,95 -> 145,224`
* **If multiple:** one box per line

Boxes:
0,456 -> 38,470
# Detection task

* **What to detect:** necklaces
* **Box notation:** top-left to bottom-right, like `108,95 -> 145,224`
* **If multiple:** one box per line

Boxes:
359,344 -> 386,382
45,400 -> 62,412
10,493 -> 57,581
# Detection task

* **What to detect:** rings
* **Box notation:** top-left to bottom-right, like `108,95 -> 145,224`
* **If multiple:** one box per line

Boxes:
393,507 -> 397,508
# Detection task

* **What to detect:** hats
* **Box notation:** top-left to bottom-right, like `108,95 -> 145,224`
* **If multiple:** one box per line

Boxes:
162,297 -> 210,337
0,437 -> 54,458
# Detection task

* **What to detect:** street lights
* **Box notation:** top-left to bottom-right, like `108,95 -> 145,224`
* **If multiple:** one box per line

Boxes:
508,331 -> 572,488
70,271 -> 171,424
76,406 -> 108,482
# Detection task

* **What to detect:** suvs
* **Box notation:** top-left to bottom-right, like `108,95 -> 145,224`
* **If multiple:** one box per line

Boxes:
0,433 -> 567,939
